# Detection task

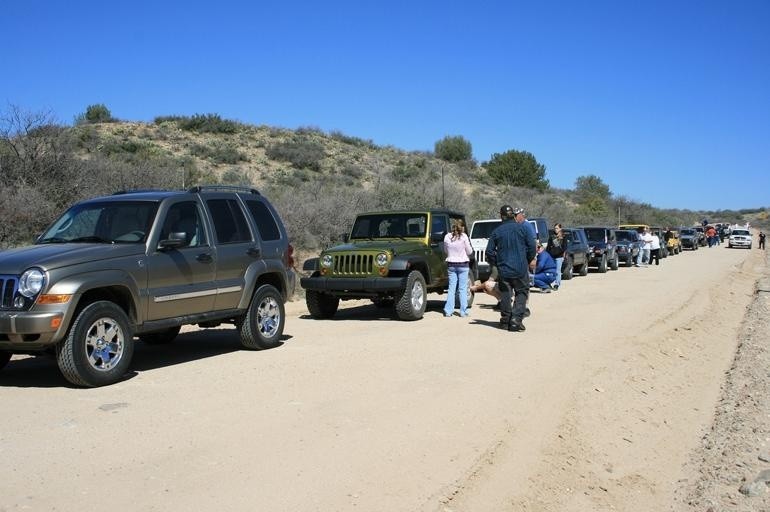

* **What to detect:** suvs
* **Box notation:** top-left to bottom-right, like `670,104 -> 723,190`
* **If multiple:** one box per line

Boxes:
0,185 -> 295,386
547,229 -> 591,279
585,226 -> 618,272
299,208 -> 477,321
467,217 -> 549,278
619,223 -> 732,259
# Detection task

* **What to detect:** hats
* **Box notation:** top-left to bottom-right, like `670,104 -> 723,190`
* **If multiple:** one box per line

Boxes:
513,207 -> 525,214
498,205 -> 513,214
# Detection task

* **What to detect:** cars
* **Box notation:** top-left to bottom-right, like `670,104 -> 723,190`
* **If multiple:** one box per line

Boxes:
616,229 -> 639,266
728,228 -> 753,250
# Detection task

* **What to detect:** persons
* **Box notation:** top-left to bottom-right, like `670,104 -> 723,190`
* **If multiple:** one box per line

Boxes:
533,223 -> 569,293
471,204 -> 536,331
703,217 -> 765,250
636,227 -> 674,267
441,218 -> 473,317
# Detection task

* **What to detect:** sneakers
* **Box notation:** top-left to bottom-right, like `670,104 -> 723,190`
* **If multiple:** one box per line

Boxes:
445,285 -> 558,332
